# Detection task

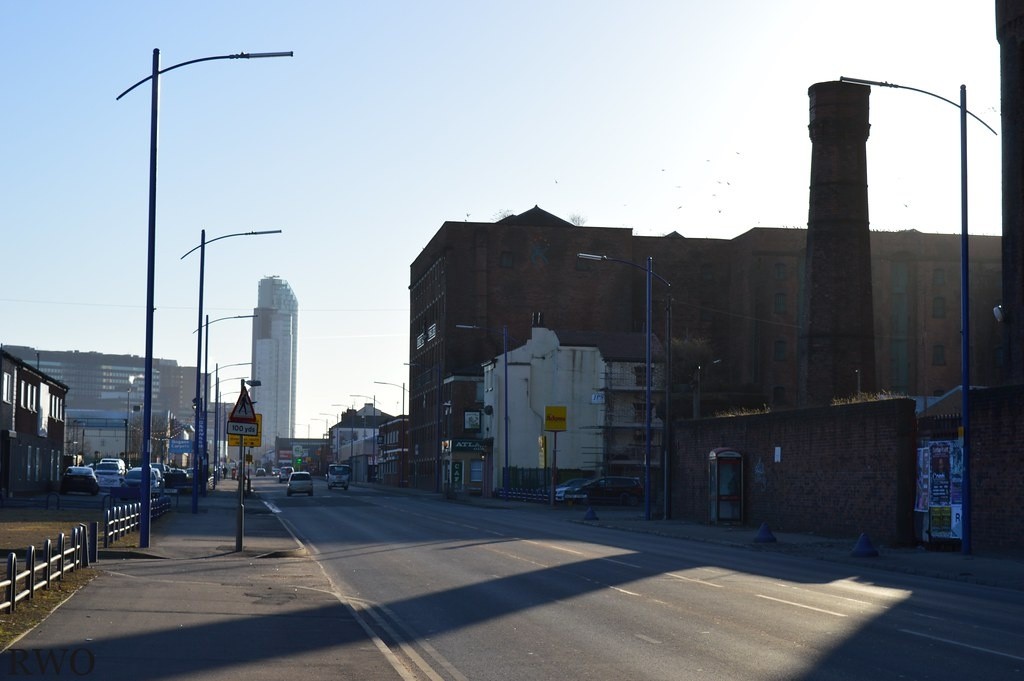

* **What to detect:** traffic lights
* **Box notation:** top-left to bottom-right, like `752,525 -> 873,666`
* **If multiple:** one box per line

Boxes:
297,457 -> 302,464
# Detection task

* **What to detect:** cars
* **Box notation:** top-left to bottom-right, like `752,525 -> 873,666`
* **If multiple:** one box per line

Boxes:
60,457 -> 194,495
537,476 -> 642,506
256,467 -> 281,477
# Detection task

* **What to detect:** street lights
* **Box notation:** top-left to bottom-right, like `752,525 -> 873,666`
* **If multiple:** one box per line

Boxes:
320,76 -> 1001,554
136,46 -> 295,548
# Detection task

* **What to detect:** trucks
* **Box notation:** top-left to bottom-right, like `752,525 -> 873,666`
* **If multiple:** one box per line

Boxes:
326,462 -> 350,490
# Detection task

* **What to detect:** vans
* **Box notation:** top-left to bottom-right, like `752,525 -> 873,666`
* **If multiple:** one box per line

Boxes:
287,471 -> 314,497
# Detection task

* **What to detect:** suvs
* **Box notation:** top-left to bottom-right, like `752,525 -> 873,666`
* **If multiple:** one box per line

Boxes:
278,467 -> 294,483
